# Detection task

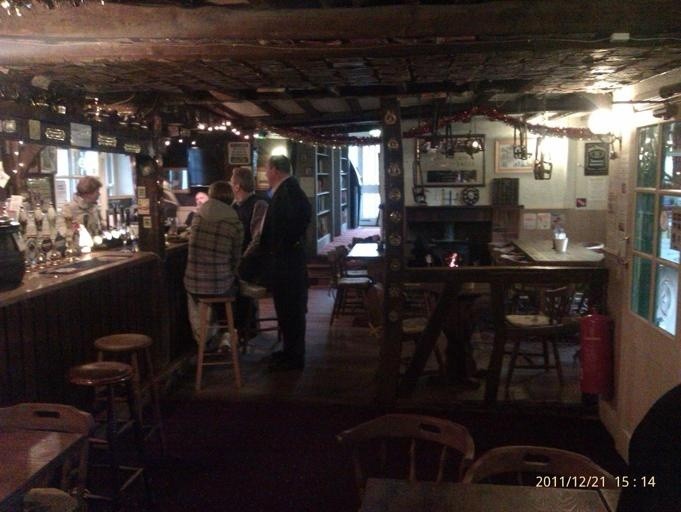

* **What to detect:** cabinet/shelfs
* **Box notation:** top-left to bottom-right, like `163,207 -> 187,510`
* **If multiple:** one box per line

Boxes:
404,204 -> 525,242
295,137 -> 334,248
330,146 -> 348,236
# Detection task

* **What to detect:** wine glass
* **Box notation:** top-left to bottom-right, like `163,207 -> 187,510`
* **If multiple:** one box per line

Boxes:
121,232 -> 131,253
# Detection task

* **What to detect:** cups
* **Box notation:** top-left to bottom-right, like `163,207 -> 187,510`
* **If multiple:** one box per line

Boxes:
553,225 -> 569,252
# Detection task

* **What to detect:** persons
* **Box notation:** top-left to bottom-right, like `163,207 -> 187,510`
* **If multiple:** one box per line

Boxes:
57,177 -> 102,242
260,155 -> 312,366
156,172 -> 180,231
183,181 -> 245,353
228,167 -> 270,337
177,188 -> 209,229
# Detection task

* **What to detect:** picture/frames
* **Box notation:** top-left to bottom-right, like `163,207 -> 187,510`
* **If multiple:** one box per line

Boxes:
493,137 -> 540,174
253,165 -> 271,191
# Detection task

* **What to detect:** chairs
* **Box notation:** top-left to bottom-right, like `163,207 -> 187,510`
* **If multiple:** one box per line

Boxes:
325,226 -> 601,403
458,438 -> 616,487
1,403 -> 92,511
335,409 -> 475,500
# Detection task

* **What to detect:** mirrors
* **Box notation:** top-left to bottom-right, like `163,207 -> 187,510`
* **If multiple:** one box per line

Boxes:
415,132 -> 486,188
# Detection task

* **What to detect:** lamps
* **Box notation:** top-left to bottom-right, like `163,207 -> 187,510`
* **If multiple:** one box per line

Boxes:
588,101 -> 630,160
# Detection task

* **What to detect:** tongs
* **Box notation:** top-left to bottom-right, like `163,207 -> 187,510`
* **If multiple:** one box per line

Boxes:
446,124 -> 456,158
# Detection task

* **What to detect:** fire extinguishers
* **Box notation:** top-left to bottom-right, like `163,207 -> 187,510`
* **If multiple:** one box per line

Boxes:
578,288 -> 616,394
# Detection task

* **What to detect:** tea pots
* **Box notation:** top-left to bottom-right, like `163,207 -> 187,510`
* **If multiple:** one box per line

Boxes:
0,86 -> 68,114
465,136 -> 486,160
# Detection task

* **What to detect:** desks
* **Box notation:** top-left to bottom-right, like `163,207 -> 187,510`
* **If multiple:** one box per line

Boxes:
1,427 -> 84,512
354,470 -> 621,512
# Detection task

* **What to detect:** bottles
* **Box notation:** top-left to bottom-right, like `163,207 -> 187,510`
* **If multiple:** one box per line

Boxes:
106,204 -> 131,233
2,204 -> 82,270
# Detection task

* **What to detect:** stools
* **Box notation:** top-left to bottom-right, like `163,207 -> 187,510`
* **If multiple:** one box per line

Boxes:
191,294 -> 241,395
94,334 -> 165,460
64,361 -> 152,511
